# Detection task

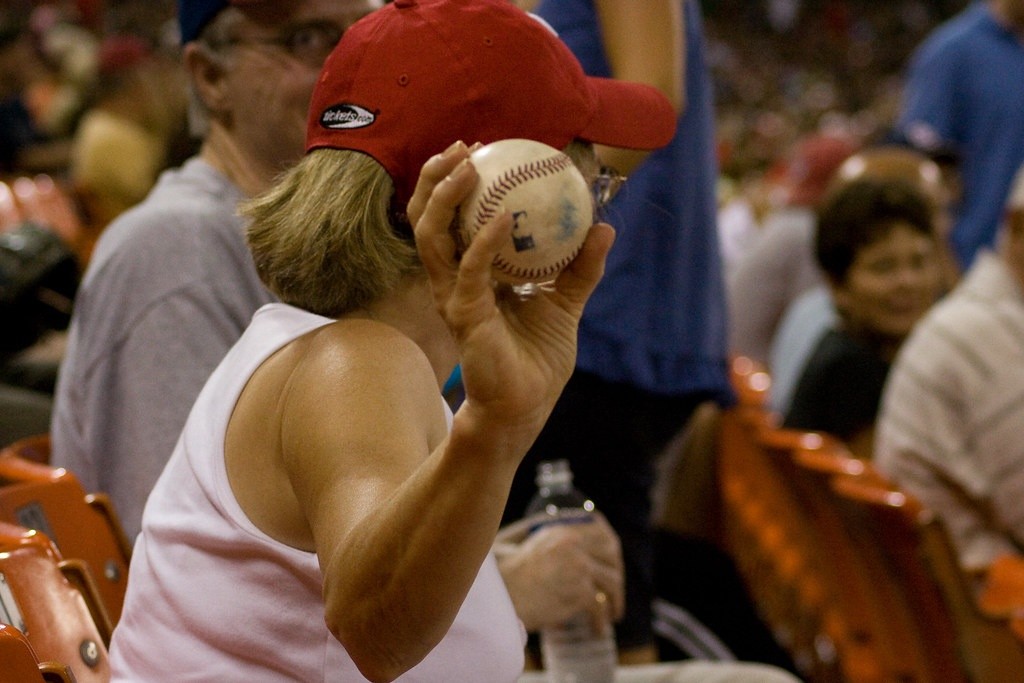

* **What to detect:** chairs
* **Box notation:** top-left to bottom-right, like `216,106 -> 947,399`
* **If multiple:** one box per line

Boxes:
719,352 -> 1023,683
0,170 -> 132,683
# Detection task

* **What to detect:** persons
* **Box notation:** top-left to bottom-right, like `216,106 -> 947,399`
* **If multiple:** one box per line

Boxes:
106,0 -> 677,683
67,36 -> 170,223
44,0 -> 383,544
770,146 -> 950,419
0,0 -> 909,665
711,127 -> 784,277
778,175 -> 937,452
871,165 -> 1024,641
489,0 -> 734,649
896,0 -> 1024,296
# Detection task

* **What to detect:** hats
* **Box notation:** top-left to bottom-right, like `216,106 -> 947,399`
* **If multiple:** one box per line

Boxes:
304,2 -> 681,226
175,0 -> 228,45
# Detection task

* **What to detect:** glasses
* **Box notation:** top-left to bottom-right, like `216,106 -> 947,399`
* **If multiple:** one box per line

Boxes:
212,20 -> 341,66
576,163 -> 627,208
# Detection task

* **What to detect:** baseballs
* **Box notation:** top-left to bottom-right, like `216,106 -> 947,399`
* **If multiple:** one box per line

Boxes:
451,138 -> 595,285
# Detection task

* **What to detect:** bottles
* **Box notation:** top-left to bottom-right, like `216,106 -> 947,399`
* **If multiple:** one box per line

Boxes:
527,459 -> 619,683
